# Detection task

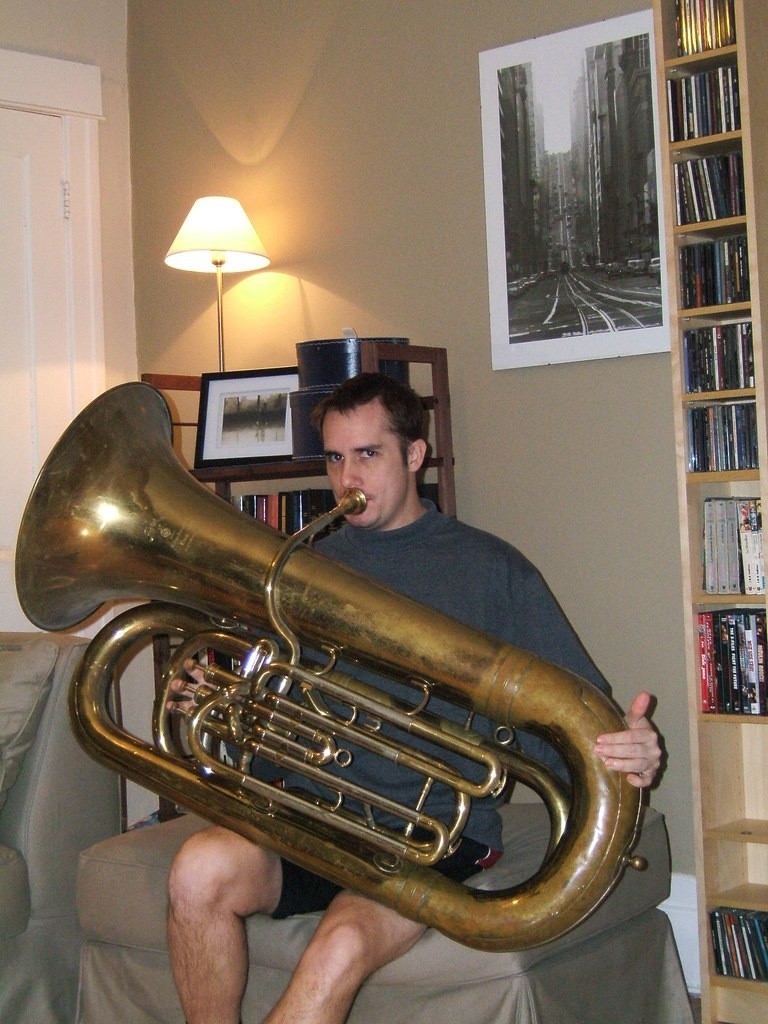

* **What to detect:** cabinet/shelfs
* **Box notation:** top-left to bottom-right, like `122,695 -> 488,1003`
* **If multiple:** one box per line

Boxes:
139,341 -> 455,804
654,0 -> 768,1024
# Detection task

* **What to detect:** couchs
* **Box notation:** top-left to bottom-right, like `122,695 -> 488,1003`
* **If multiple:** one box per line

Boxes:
0,633 -> 122,1024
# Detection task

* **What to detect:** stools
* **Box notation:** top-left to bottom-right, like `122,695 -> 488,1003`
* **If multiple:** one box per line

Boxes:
75,802 -> 695,1024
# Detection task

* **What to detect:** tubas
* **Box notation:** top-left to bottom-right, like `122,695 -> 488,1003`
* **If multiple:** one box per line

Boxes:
16,382 -> 648,954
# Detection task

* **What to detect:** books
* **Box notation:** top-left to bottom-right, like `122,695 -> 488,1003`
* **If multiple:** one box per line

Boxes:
198,489 -> 338,693
168,373 -> 662,1022
709,908 -> 767,983
668,0 -> 768,714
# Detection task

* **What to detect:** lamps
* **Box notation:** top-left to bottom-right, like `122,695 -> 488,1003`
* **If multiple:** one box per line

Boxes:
164,196 -> 270,372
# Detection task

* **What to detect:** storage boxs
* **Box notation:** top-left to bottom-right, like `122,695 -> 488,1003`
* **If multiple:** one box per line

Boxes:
289,328 -> 410,461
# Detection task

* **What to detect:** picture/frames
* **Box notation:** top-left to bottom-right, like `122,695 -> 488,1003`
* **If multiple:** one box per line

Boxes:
194,366 -> 299,468
